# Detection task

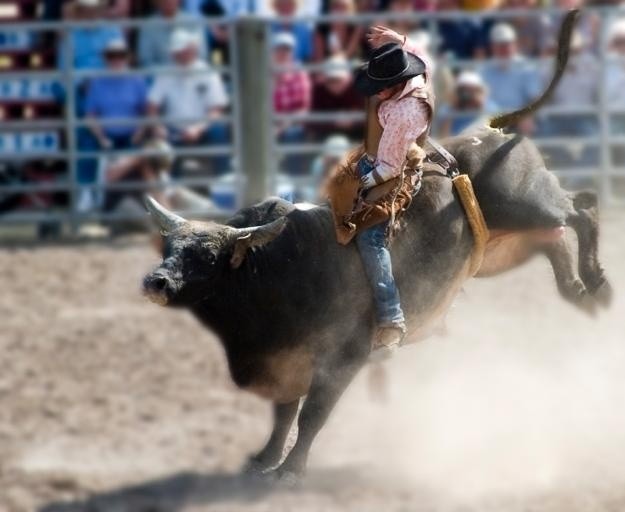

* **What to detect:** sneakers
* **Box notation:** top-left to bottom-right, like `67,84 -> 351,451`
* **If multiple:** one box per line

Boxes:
370,325 -> 406,360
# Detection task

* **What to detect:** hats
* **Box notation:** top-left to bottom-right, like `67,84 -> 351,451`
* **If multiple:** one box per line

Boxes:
453,22 -> 519,91
99,29 -> 352,80
354,43 -> 426,97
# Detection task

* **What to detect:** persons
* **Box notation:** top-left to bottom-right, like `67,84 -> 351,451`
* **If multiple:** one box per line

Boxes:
322,26 -> 435,365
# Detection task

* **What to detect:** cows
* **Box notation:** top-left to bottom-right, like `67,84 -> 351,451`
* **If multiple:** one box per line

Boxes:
138,7 -> 615,491
